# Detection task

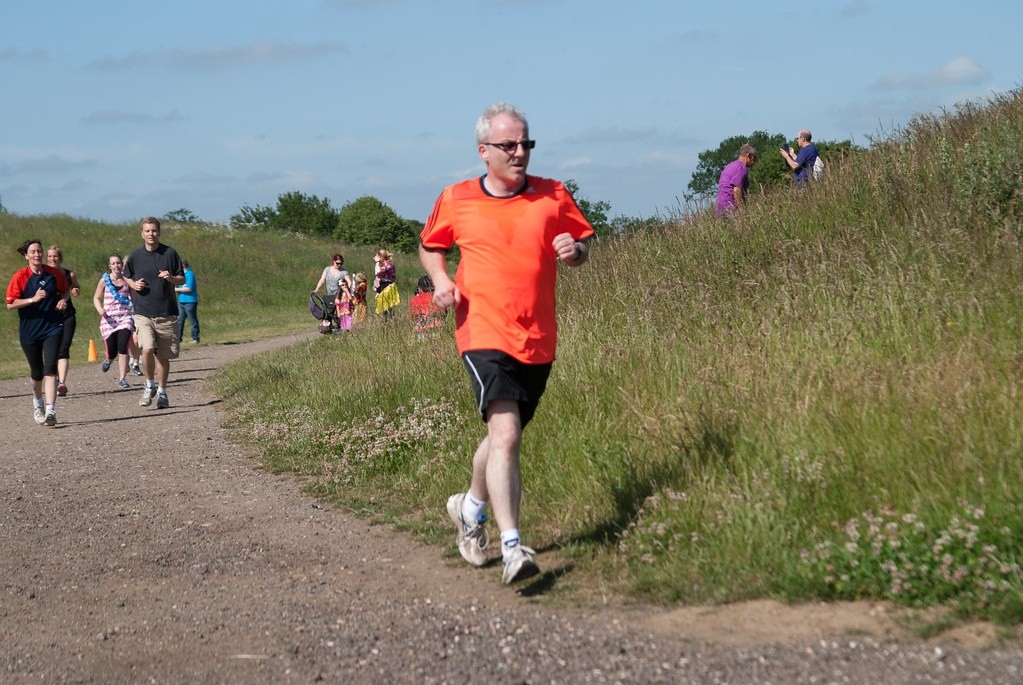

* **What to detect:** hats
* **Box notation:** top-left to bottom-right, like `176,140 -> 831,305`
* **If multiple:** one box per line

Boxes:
418,276 -> 435,290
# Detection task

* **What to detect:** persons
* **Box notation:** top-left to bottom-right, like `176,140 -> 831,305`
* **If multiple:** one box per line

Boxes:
314,254 -> 352,331
373,250 -> 400,324
175,260 -> 200,344
715,144 -> 757,215
410,276 -> 446,339
419,105 -> 595,585
813,156 -> 824,178
5,240 -> 70,426
353,273 -> 367,322
47,245 -> 81,397
779,130 -> 819,184
335,279 -> 354,332
123,255 -> 143,376
93,253 -> 134,389
122,217 -> 185,409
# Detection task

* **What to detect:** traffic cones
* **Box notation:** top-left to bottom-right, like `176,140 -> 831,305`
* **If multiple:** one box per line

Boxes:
88,339 -> 99,363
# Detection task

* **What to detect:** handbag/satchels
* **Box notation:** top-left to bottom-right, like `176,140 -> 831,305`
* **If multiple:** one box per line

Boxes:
318,319 -> 331,333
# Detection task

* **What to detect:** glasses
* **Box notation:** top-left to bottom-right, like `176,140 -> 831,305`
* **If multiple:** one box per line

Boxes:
335,261 -> 343,265
483,140 -> 535,151
747,152 -> 757,160
796,138 -> 799,140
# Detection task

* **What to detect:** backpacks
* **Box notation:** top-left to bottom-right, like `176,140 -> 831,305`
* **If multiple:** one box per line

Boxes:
801,155 -> 824,182
309,290 -> 328,320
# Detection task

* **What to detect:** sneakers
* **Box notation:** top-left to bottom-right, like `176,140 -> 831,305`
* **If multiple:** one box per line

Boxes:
101,359 -> 113,372
57,384 -> 67,397
446,491 -> 490,567
45,409 -> 57,426
500,542 -> 540,585
119,379 -> 129,389
139,383 -> 157,406
125,366 -> 130,372
133,365 -> 142,376
129,356 -> 134,365
157,392 -> 168,408
33,399 -> 46,425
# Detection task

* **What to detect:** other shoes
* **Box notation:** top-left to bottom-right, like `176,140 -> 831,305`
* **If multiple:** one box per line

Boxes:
191,340 -> 198,344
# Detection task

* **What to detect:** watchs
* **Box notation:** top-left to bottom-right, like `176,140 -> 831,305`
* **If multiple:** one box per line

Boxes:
572,244 -> 581,261
29,298 -> 32,303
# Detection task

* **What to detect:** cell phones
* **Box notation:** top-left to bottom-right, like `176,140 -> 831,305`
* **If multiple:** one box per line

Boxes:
783,144 -> 790,154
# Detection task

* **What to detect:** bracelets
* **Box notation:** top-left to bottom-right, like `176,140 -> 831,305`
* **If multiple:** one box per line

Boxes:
375,261 -> 381,263
